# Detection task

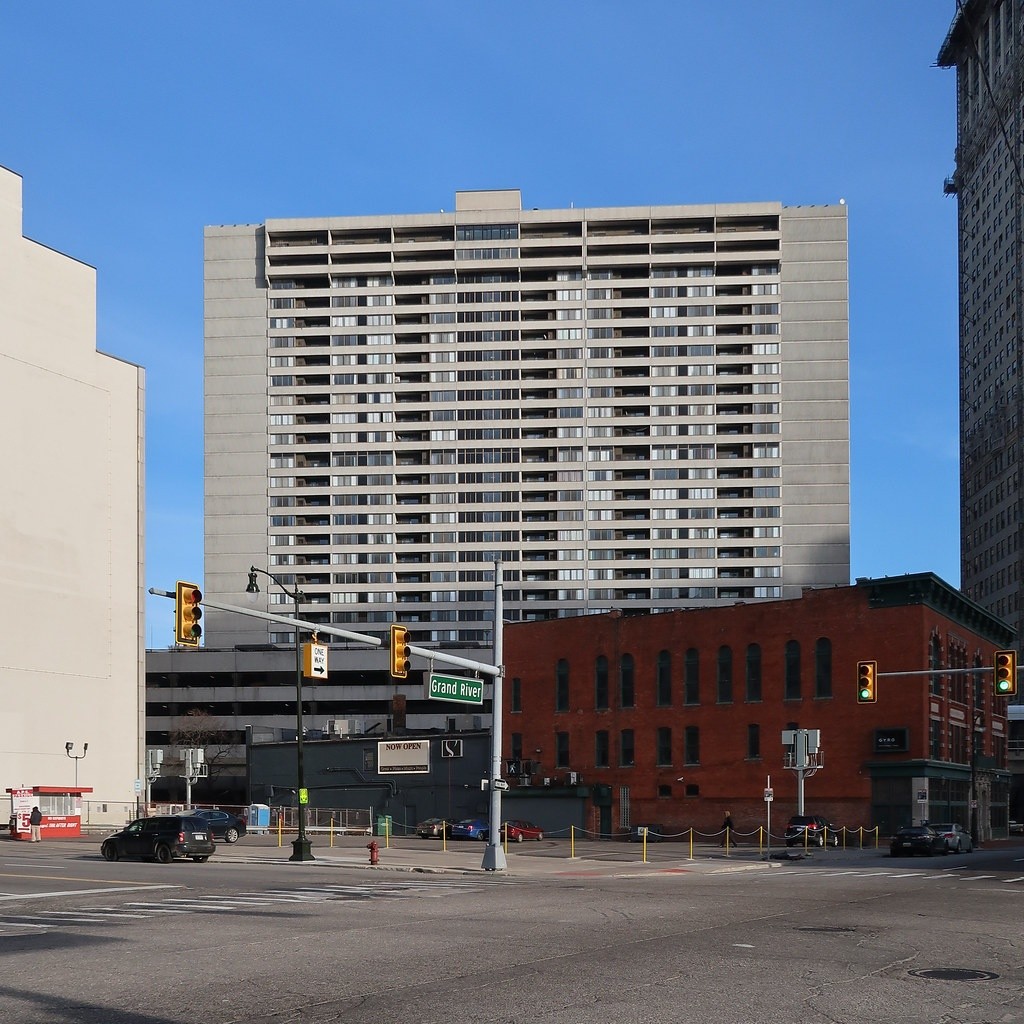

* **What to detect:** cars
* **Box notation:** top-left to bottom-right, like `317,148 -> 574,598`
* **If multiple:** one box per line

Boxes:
889,824 -> 949,857
927,823 -> 973,854
416,817 -> 462,839
501,819 -> 544,843
452,818 -> 489,841
172,809 -> 247,843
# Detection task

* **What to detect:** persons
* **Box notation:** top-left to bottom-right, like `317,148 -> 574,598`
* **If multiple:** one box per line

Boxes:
719,811 -> 738,848
29,807 -> 42,843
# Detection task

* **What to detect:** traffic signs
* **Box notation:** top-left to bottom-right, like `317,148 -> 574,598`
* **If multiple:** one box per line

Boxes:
310,643 -> 328,679
493,778 -> 510,792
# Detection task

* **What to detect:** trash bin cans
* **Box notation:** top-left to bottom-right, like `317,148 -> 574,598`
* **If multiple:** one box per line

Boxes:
372,815 -> 392,836
250,804 -> 270,835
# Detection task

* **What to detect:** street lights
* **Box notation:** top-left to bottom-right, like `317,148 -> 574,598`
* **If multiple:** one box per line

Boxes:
246,565 -> 315,861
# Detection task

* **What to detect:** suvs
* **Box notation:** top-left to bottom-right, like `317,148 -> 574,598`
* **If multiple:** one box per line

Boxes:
786,815 -> 840,847
101,815 -> 216,863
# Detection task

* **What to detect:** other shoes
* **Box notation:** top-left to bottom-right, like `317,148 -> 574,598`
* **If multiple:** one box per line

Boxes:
719,845 -> 723,848
29,841 -> 35,843
37,840 -> 40,842
732,845 -> 737,848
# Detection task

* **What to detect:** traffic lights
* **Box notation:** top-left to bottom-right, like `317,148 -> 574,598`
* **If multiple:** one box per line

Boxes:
506,760 -> 538,775
175,581 -> 202,647
857,660 -> 877,704
994,650 -> 1018,696
390,624 -> 411,678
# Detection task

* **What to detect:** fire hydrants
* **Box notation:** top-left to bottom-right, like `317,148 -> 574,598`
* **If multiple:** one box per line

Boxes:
366,840 -> 379,865
